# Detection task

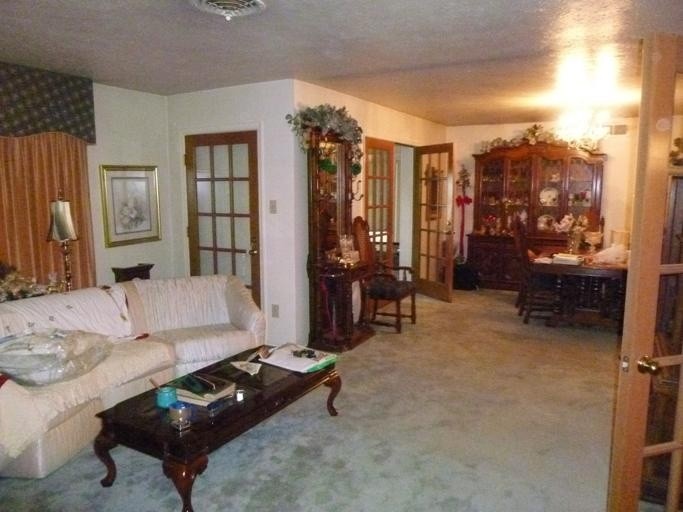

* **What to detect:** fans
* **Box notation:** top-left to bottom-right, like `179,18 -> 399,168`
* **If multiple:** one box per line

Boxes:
189,0 -> 268,22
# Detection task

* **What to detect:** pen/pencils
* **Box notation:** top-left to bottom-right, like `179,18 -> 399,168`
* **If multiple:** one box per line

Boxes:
192,375 -> 216,391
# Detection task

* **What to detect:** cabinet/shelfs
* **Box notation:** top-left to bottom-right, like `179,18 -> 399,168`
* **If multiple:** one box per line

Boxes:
465,123 -> 607,292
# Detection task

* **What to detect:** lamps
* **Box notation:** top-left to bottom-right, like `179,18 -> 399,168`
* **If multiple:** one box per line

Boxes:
45,198 -> 79,292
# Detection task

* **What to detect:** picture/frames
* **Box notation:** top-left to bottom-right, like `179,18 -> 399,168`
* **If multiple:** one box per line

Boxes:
97,162 -> 162,249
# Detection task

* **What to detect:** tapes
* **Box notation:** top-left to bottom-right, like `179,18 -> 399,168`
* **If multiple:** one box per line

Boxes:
168,402 -> 193,418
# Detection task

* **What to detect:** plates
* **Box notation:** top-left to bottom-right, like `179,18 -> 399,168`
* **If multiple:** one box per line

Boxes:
537,187 -> 560,204
535,214 -> 556,231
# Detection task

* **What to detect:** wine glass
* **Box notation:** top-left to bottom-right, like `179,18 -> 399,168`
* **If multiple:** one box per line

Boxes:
582,232 -> 602,255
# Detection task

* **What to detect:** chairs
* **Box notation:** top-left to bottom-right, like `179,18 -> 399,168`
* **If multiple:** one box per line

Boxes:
351,215 -> 416,334
514,219 -> 629,334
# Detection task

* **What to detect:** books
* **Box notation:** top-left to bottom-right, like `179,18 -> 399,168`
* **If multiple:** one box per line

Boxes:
257,340 -> 336,374
174,393 -> 216,407
551,251 -> 582,266
168,372 -> 236,401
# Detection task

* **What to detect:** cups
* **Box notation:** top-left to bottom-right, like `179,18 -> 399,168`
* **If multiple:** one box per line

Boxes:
155,386 -> 177,409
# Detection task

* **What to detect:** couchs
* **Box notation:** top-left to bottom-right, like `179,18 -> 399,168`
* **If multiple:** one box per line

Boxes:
0,276 -> 266,480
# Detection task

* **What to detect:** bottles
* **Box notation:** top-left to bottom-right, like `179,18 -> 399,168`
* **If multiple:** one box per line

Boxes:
236,389 -> 245,402
